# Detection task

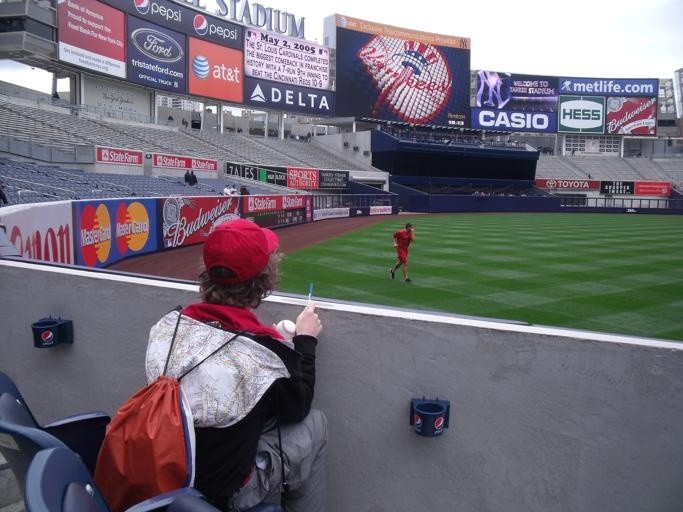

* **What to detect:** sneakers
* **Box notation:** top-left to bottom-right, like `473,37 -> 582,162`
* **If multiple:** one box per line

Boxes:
403,278 -> 413,283
390,268 -> 395,279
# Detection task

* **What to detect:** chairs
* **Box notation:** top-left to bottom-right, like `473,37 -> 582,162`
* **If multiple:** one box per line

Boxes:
0,370 -> 224,511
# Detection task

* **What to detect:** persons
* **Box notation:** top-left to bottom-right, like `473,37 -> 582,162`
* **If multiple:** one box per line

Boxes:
0,177 -> 11,205
223,183 -> 232,196
230,184 -> 239,195
190,171 -> 198,187
391,222 -> 415,282
184,169 -> 192,184
144,219 -> 330,511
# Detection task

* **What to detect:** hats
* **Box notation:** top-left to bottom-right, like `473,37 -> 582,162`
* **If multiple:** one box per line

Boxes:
203,218 -> 280,284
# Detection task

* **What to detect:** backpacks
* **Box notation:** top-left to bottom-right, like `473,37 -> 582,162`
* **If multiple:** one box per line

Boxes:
93,308 -> 245,511
